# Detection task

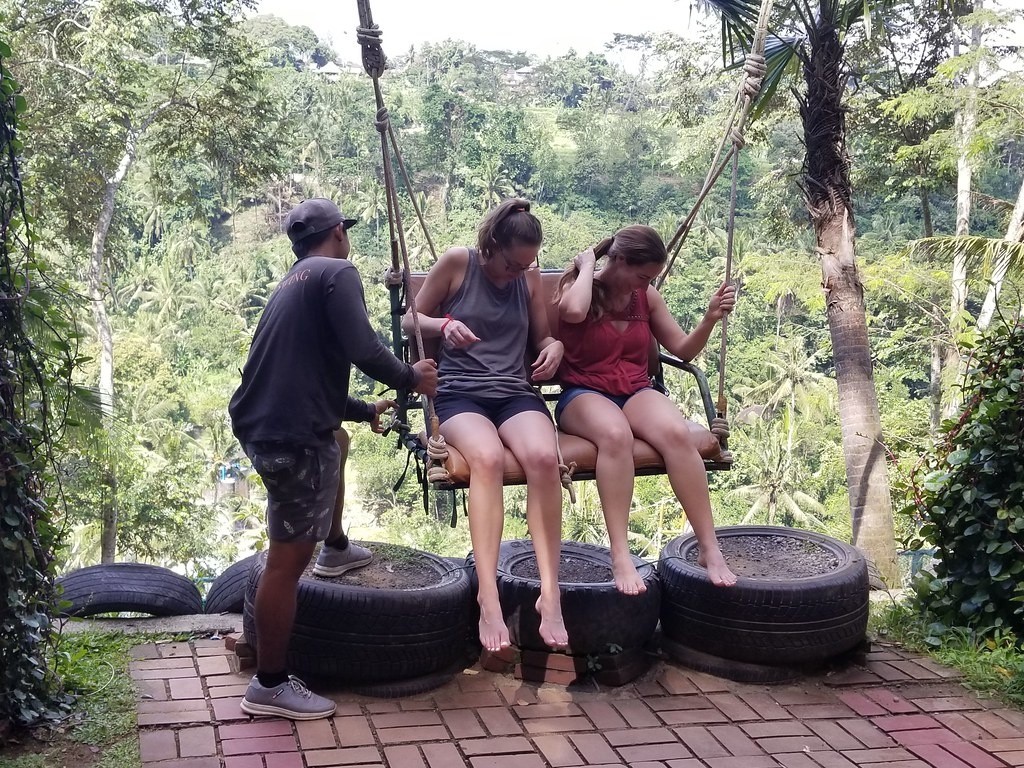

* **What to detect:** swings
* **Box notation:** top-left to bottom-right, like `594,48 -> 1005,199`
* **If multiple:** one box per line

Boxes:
353,0 -> 777,491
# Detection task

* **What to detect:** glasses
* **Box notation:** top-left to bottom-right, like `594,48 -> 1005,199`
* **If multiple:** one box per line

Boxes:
499,251 -> 541,273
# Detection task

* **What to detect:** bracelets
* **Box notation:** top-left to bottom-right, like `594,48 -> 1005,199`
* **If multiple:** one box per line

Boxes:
441,314 -> 452,337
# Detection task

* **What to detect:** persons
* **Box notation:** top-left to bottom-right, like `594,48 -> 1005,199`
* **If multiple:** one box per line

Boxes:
403,199 -> 570,650
228,198 -> 438,721
553,225 -> 736,595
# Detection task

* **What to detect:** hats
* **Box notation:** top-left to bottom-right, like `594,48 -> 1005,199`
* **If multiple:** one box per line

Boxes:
288,197 -> 358,246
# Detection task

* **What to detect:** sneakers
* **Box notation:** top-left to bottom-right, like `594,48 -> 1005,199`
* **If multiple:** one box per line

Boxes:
312,543 -> 373,576
240,676 -> 337,721
